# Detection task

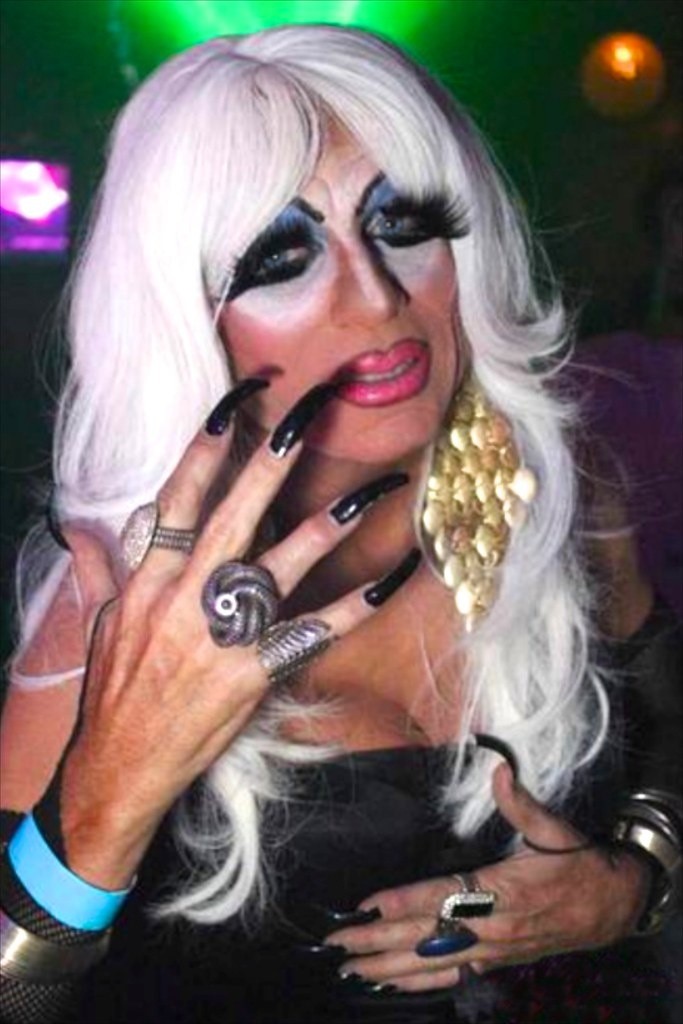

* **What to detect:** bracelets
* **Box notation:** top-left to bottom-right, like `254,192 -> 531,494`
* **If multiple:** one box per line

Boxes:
0,911 -> 112,985
4,809 -> 138,933
607,780 -> 683,937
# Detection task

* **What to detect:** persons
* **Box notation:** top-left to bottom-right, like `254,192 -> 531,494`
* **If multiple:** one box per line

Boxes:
0,24 -> 683,1024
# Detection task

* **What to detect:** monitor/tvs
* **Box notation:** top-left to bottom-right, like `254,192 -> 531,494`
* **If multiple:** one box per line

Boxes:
0,157 -> 73,253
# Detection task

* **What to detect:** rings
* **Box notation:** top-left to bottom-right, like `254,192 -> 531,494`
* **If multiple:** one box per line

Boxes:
441,870 -> 495,920
202,562 -> 280,650
257,615 -> 338,682
116,504 -> 195,576
456,961 -> 481,988
415,918 -> 477,957
